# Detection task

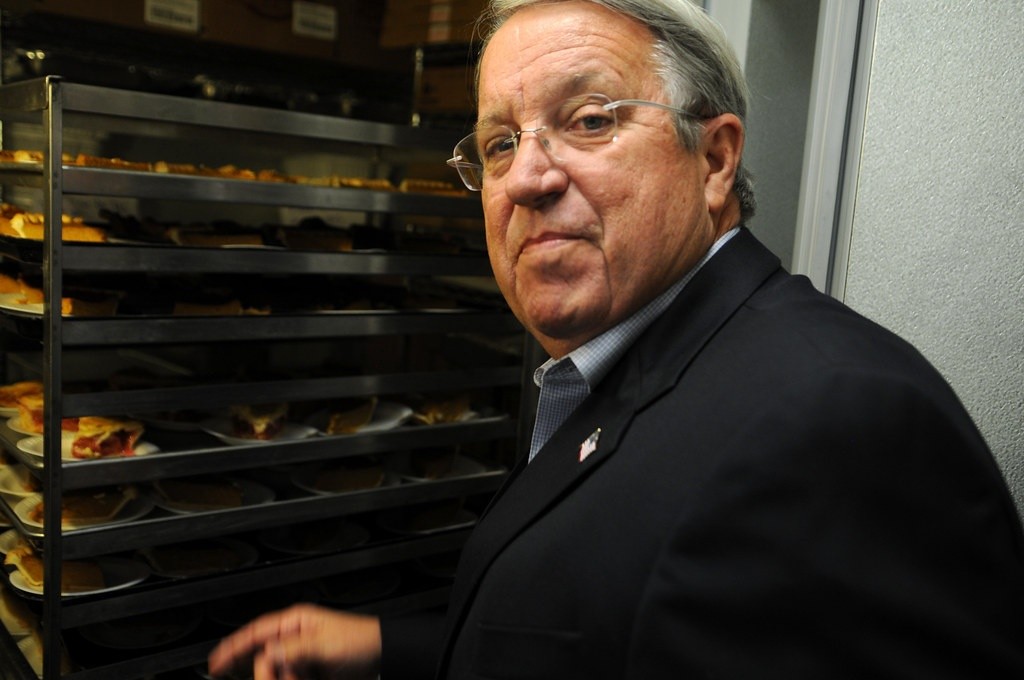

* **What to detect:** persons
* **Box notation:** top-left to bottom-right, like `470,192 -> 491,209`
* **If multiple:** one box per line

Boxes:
210,0 -> 1024,680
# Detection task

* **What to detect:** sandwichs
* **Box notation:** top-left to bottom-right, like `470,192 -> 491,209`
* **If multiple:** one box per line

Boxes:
0,381 -> 472,460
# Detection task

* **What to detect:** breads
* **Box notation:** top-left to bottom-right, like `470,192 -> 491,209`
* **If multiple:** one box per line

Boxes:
0,150 -> 468,318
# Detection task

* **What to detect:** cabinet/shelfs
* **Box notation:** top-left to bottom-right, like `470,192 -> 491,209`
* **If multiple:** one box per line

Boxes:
0,45 -> 549,680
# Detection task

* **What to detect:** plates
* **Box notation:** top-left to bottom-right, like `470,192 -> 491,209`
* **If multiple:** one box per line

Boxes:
0,143 -> 513,600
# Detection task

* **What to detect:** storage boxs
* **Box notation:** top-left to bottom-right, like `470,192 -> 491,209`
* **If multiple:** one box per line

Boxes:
12,0 -> 341,64
380,0 -> 490,44
419,65 -> 475,108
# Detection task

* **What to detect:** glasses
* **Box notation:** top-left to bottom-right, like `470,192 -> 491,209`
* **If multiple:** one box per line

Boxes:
446,91 -> 702,192
